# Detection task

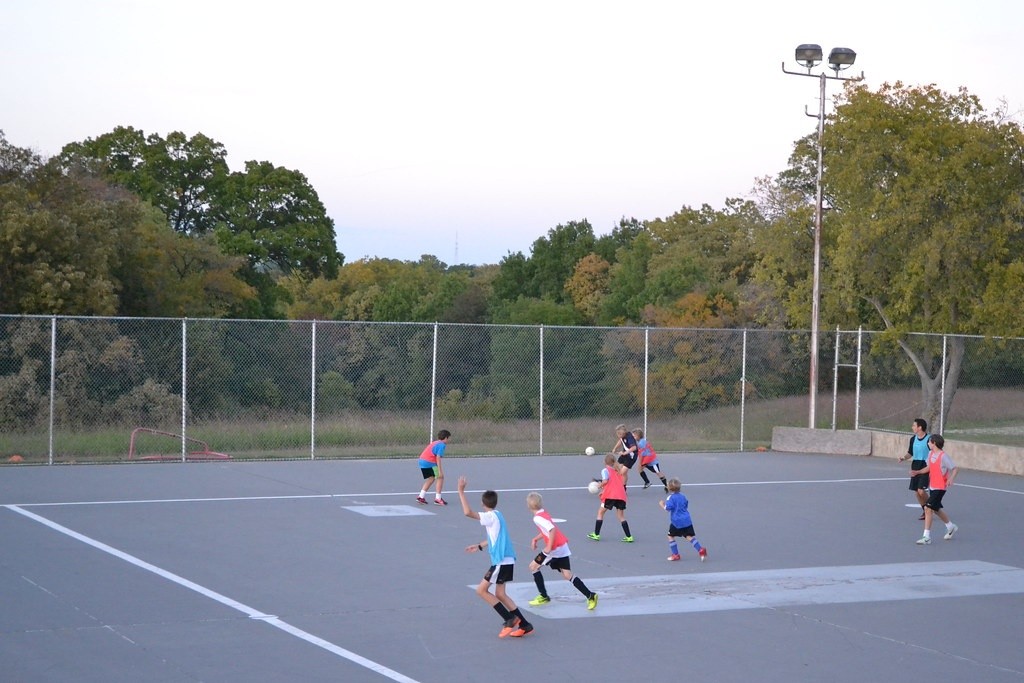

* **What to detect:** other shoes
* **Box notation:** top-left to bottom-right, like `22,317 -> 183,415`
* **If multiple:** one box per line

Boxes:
919,514 -> 925,520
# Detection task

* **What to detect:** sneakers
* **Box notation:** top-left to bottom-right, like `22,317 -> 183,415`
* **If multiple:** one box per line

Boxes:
621,536 -> 634,542
643,481 -> 652,489
667,554 -> 680,561
529,595 -> 551,605
664,486 -> 668,492
416,496 -> 428,504
499,615 -> 521,639
916,536 -> 931,544
944,524 -> 958,539
587,592 -> 598,610
699,547 -> 707,561
510,623 -> 535,637
434,498 -> 447,505
587,532 -> 600,541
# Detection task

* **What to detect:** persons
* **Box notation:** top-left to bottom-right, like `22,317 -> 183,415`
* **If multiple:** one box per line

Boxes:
897,418 -> 932,520
612,425 -> 666,494
659,479 -> 707,562
527,492 -> 598,611
586,453 -> 635,542
458,476 -> 535,637
416,429 -> 449,505
911,434 -> 961,544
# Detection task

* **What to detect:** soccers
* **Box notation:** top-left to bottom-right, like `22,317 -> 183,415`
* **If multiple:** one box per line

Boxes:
588,482 -> 600,493
585,447 -> 595,456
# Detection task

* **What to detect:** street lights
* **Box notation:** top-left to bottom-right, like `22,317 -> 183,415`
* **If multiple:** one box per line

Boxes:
782,43 -> 863,428
